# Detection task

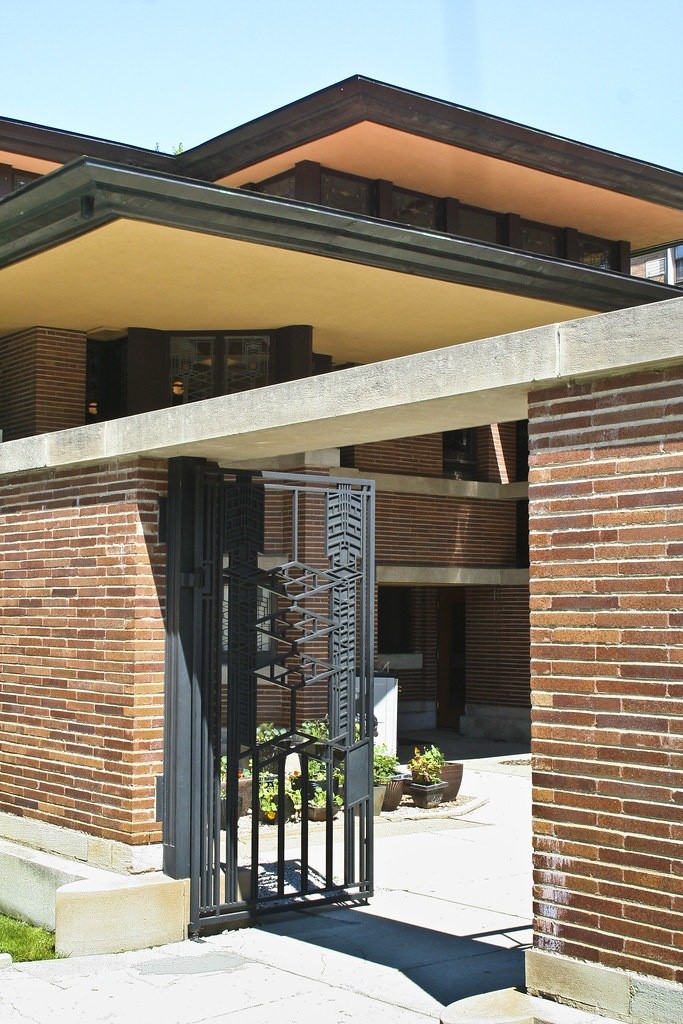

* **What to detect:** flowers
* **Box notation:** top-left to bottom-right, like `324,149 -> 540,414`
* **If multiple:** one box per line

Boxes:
259,784 -> 282,820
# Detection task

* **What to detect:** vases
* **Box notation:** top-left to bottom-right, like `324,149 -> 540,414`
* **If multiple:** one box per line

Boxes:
259,794 -> 295,824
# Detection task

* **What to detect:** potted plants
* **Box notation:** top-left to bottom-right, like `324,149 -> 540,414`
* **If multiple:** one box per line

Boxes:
217,717 -> 463,830
